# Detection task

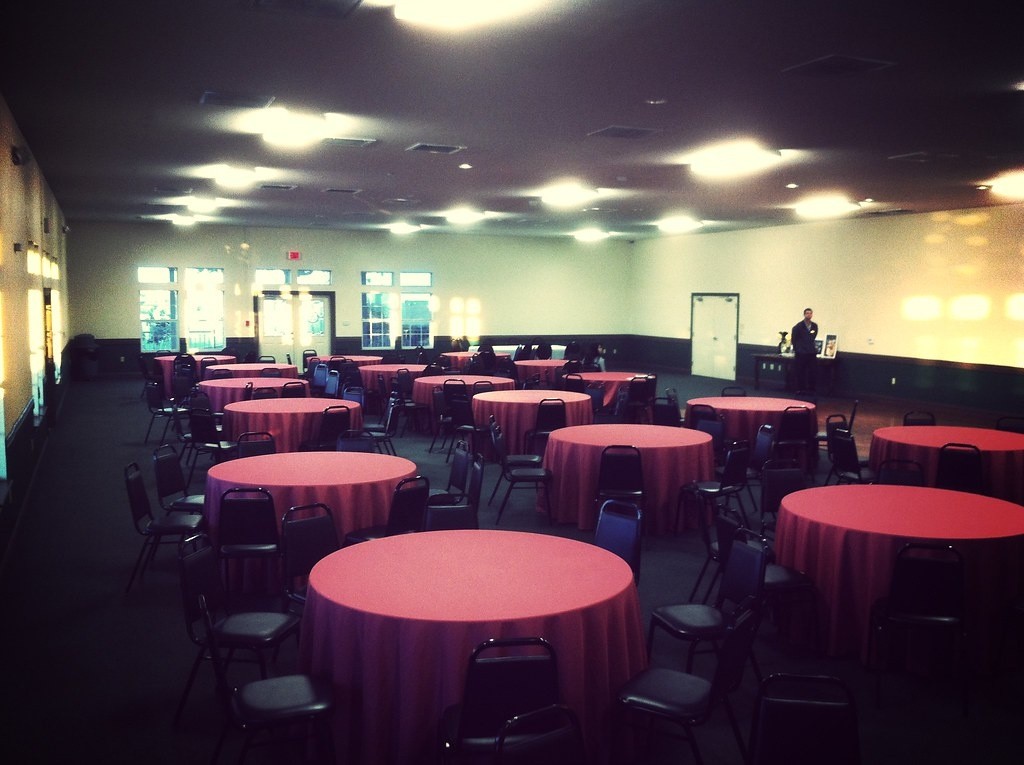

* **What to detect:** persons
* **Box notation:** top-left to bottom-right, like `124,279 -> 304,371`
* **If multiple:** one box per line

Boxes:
580,341 -> 607,373
791,308 -> 819,396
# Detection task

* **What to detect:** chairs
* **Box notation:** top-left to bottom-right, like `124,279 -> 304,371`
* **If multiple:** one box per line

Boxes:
120,339 -> 1024,764
